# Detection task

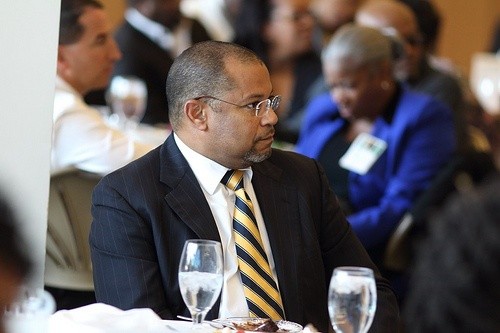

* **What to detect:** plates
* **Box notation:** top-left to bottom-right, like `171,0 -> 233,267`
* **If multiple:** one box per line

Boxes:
205,318 -> 302,333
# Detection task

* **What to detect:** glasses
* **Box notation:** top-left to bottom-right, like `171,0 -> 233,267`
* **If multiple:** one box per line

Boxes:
194,95 -> 281,116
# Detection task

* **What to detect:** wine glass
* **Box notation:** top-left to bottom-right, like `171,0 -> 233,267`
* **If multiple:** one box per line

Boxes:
110,76 -> 147,128
178,240 -> 223,329
328,266 -> 378,333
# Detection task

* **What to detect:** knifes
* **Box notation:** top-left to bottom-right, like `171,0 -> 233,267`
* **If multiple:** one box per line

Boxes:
177,315 -> 256,332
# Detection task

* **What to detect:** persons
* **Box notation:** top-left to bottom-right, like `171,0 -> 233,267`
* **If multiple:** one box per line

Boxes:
88,41 -> 401,333
294,22 -> 465,256
106,0 -> 500,163
43,0 -> 171,175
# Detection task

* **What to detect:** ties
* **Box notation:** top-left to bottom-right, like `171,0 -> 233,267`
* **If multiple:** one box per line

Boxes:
221,170 -> 285,322
